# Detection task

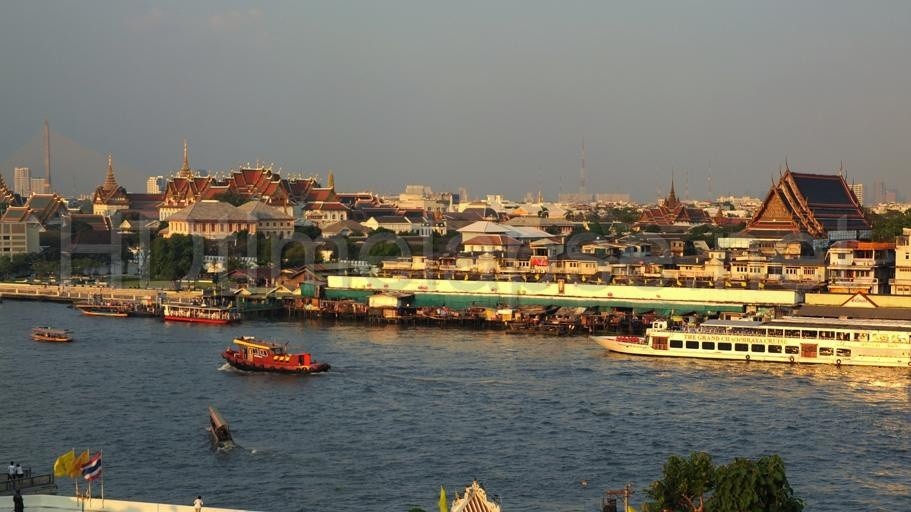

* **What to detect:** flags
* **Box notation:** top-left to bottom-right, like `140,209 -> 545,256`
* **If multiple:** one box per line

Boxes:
54,451 -> 75,477
79,451 -> 103,480
67,450 -> 90,479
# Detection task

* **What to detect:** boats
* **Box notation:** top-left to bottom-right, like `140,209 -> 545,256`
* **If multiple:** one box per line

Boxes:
221,335 -> 331,374
31,326 -> 74,342
76,297 -> 242,324
207,405 -> 233,446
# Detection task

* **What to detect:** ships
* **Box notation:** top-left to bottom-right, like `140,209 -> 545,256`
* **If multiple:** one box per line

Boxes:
590,318 -> 911,368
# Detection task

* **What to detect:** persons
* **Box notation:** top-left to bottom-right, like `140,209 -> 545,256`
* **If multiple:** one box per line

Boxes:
193,495 -> 203,512
16,464 -> 24,485
13,488 -> 24,512
7,461 -> 16,490
